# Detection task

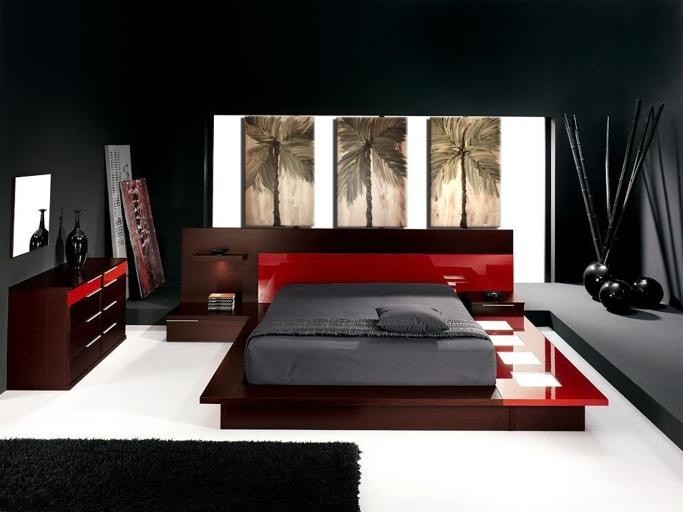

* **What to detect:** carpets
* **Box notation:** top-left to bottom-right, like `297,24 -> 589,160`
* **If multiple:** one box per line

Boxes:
0,438 -> 361,512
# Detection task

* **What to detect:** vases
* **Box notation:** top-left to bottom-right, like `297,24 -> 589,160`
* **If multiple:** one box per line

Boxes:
65,209 -> 88,272
584,262 -> 664,314
30,210 -> 48,251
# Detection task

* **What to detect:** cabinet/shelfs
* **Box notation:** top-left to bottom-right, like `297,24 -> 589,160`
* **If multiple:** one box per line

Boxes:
6,258 -> 128,390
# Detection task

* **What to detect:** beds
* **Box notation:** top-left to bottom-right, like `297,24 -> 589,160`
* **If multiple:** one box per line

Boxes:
165,228 -> 608,431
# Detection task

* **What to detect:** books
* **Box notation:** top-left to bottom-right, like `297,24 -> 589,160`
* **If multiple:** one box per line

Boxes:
207,293 -> 235,311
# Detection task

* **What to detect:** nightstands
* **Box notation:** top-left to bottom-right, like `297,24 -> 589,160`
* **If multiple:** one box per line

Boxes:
463,291 -> 525,316
165,302 -> 248,342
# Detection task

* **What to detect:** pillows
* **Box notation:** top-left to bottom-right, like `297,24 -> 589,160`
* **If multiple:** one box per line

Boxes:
375,308 -> 449,336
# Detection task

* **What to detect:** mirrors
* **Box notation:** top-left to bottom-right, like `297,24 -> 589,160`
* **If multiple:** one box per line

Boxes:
10,173 -> 52,259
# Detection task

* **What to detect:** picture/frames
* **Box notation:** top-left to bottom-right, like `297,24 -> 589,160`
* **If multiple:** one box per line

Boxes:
427,117 -> 502,230
334,117 -> 408,229
242,115 -> 315,230
